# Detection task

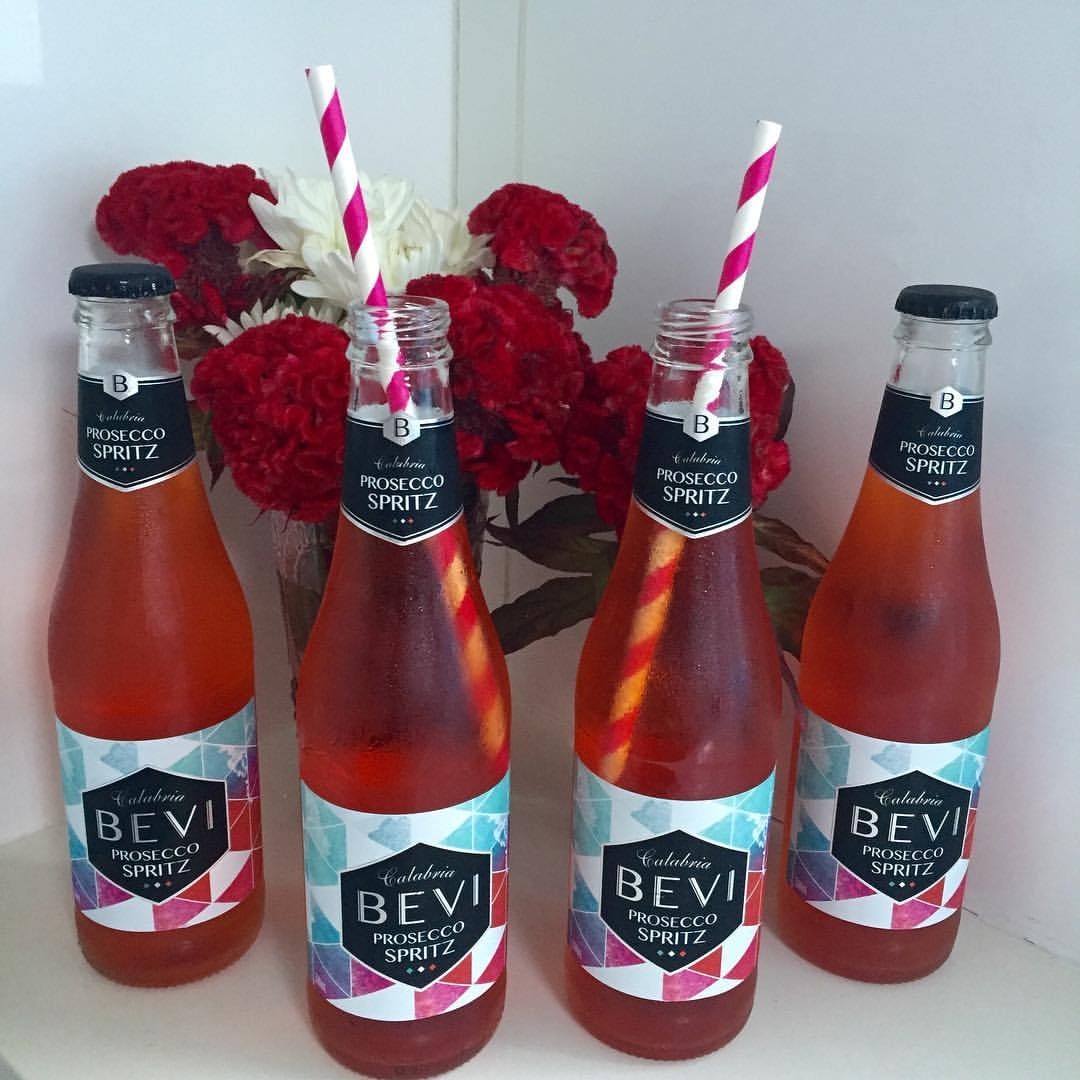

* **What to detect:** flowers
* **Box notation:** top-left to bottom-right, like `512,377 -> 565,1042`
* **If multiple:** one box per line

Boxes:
95,155 -> 835,653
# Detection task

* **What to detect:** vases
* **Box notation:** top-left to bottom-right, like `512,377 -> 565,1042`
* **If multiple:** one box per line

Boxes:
269,506 -> 486,720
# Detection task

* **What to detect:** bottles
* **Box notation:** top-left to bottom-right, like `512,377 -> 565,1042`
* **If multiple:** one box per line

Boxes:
778,284 -> 1000,982
46,262 -> 266,995
568,298 -> 788,1062
298,294 -> 509,1080
